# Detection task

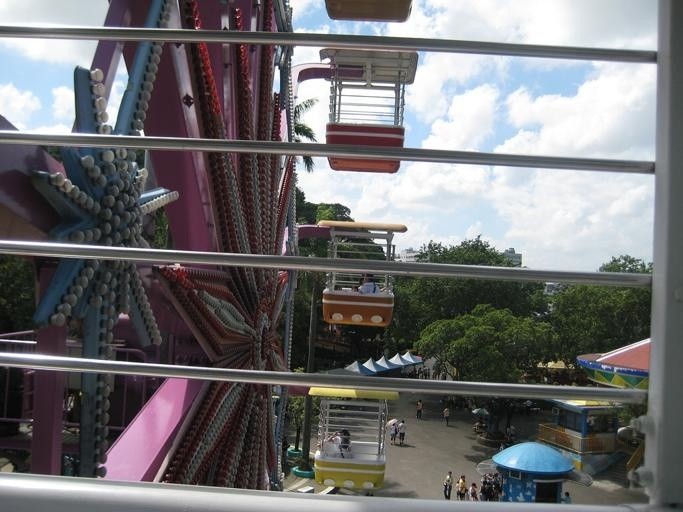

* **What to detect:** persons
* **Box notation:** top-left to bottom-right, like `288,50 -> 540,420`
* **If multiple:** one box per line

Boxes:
586,415 -> 594,433
443,471 -> 453,499
440,394 -> 525,416
497,424 -> 516,452
455,472 -> 500,501
472,417 -> 486,431
334,274 -> 386,293
398,420 -> 406,446
525,400 -> 532,415
561,492 -> 571,503
416,399 -> 424,419
418,365 -> 447,380
390,423 -> 398,445
328,429 -> 351,459
443,407 -> 450,426
